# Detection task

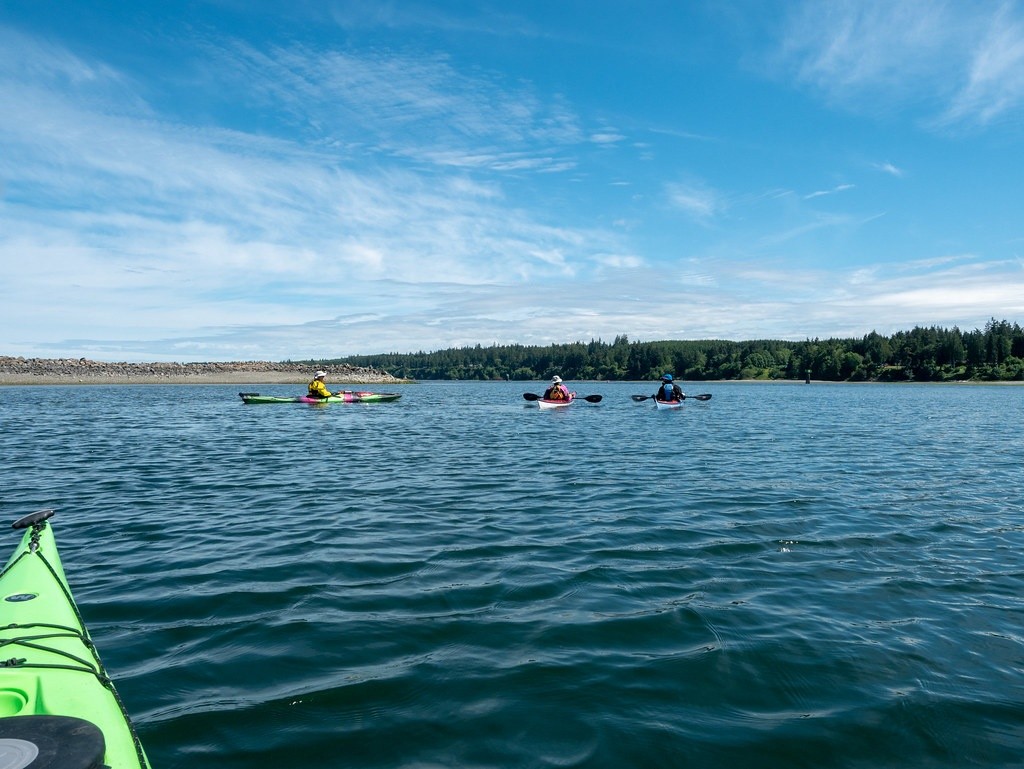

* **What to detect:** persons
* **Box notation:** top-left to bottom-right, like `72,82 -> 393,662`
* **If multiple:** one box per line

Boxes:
308,371 -> 331,398
544,375 -> 576,404
657,374 -> 686,402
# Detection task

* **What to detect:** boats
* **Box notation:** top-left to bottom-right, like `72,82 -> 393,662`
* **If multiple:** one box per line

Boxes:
239,390 -> 404,404
536,390 -> 578,409
1,514 -> 150,769
654,393 -> 686,409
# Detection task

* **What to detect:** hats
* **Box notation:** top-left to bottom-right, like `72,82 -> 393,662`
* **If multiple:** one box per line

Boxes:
313,371 -> 327,380
550,375 -> 563,384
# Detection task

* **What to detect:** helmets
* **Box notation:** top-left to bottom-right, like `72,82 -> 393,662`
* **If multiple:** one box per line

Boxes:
663,374 -> 672,380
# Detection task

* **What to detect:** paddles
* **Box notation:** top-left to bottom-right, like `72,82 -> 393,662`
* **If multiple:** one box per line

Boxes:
522,393 -> 602,403
629,393 -> 711,401
